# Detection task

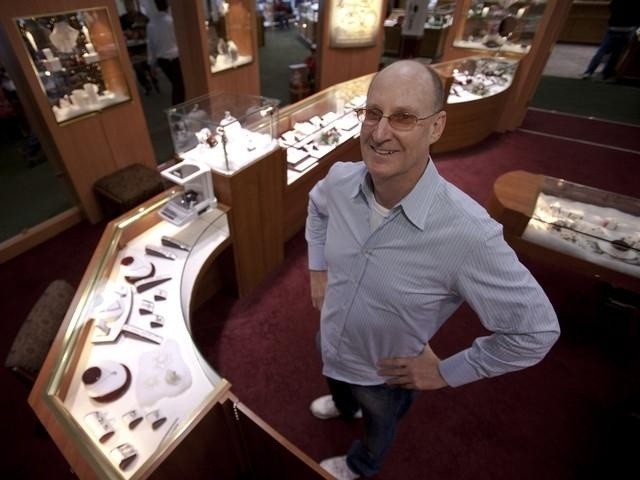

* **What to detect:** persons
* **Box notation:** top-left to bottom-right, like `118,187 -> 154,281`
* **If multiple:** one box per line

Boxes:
119,0 -> 164,95
583,0 -> 640,82
304,60 -> 561,480
304,44 -> 317,88
274,0 -> 288,29
146,0 -> 185,105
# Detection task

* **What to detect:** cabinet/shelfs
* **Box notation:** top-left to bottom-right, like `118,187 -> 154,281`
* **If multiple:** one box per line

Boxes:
12,7 -> 133,125
489,168 -> 640,291
200,0 -> 256,75
453,1 -> 552,54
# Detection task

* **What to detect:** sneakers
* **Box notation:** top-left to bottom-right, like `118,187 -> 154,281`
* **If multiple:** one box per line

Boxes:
310,395 -> 362,419
320,456 -> 361,480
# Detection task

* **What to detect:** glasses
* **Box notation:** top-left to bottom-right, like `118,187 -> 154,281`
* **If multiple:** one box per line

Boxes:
354,101 -> 441,131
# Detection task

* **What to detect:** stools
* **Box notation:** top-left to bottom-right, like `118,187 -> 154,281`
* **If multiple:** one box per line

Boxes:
93,163 -> 174,220
